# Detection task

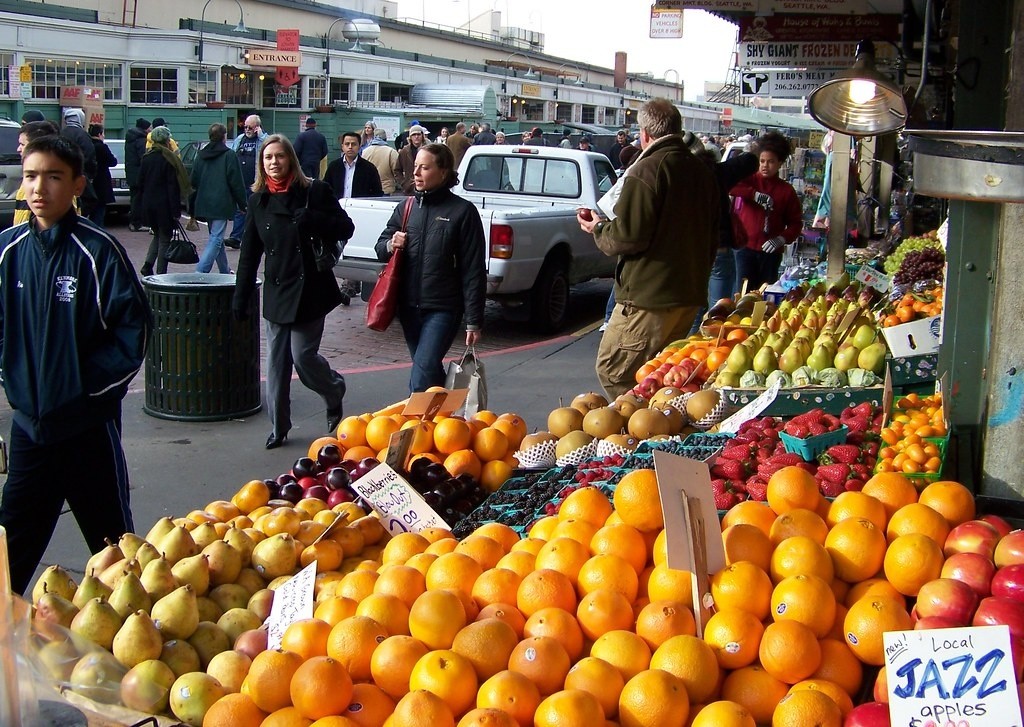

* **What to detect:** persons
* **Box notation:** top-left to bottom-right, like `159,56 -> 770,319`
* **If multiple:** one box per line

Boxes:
394,120 -> 472,195
465,122 -> 510,145
559,128 -> 574,149
22,108 -> 118,228
223,114 -> 269,250
12,121 -> 81,227
354,120 -> 400,196
0,138 -> 154,597
293,118 -> 328,180
373,144 -> 489,399
186,123 -> 247,275
576,137 -> 592,151
576,98 -> 729,402
124,117 -> 198,278
231,135 -> 355,449
516,127 -> 551,146
598,129 -> 644,333
680,128 -> 803,337
322,132 -> 384,200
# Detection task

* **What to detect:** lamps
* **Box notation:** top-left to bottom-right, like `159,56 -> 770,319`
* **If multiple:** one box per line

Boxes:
809,36 -> 954,138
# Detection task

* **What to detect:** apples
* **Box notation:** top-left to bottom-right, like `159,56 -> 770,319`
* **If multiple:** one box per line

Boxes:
842,515 -> 1024,727
626,358 -> 712,399
698,292 -> 776,336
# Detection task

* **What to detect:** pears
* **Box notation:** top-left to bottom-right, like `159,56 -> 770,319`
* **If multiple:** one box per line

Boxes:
519,387 -> 721,465
23,514 -> 299,726
715,270 -> 887,388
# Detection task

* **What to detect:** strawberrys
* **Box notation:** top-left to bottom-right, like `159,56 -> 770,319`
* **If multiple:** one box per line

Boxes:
709,400 -> 884,510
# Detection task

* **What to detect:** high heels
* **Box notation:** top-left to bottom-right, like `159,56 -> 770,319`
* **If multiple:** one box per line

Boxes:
326,401 -> 343,432
266,432 -> 288,448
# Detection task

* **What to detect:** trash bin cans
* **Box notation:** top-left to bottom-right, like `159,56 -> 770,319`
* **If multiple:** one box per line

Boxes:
140,273 -> 262,422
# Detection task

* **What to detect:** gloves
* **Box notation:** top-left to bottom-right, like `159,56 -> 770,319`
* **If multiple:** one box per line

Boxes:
755,192 -> 774,212
762,236 -> 785,253
234,308 -> 248,321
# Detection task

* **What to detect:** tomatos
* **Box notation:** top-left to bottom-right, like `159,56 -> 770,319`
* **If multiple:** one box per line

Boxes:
877,392 -> 946,488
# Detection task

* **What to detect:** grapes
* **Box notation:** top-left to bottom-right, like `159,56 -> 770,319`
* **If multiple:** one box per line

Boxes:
883,233 -> 945,285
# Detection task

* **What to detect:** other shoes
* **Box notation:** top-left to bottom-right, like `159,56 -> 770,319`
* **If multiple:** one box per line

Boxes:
141,264 -> 153,278
598,323 -> 609,332
224,238 -> 240,249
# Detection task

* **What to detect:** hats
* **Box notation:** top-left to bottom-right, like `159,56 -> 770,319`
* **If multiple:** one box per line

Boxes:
619,145 -> 643,168
22,110 -> 44,122
151,126 -> 170,142
580,136 -> 590,142
374,129 -> 388,140
136,118 -> 152,130
152,118 -> 169,127
681,130 -> 705,155
306,118 -> 316,127
409,125 -> 430,136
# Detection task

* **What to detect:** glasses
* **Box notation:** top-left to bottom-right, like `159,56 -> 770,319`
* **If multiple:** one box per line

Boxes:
244,125 -> 257,130
22,122 -> 27,126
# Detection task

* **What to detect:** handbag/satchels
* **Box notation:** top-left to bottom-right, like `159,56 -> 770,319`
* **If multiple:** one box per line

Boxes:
444,344 -> 488,420
163,220 -> 199,264
366,196 -> 414,332
297,181 -> 340,274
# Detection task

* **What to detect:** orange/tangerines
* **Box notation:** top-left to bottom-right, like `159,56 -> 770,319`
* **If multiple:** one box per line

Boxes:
633,328 -> 748,385
209,467 -> 975,727
883,286 -> 942,328
309,386 -> 525,491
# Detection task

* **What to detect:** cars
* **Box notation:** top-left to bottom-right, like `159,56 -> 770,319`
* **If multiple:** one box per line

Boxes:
103,138 -> 131,214
599,169 -> 625,198
178,140 -> 235,214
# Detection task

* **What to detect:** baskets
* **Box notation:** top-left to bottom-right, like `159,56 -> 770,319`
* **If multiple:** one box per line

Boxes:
446,397 -> 951,537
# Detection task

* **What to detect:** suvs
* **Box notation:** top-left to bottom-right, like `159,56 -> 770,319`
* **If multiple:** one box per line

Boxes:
0,116 -> 23,214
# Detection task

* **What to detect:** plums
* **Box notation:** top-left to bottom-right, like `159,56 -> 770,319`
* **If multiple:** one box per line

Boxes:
263,445 -> 486,528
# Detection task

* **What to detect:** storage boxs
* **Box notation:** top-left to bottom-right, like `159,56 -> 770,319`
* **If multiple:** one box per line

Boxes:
62,107 -> 104,138
60,84 -> 102,107
455,251 -> 945,541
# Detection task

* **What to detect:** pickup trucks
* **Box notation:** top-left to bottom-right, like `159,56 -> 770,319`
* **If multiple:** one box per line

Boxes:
331,145 -> 618,336
